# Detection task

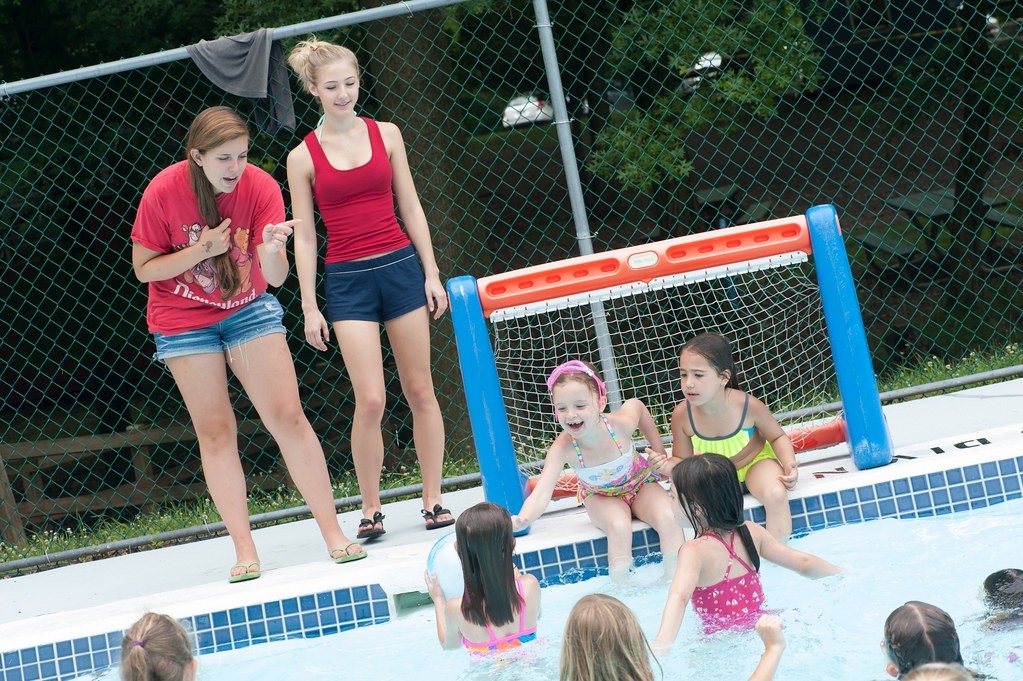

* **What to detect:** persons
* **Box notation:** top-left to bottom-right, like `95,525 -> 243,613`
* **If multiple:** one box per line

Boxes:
130,106 -> 369,584
119,613 -> 197,681
287,35 -> 454,537
425,333 -> 1023,681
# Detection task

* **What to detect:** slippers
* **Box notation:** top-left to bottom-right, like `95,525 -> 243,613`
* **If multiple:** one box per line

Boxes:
357,511 -> 386,539
329,542 -> 366,563
228,562 -> 261,583
421,504 -> 455,530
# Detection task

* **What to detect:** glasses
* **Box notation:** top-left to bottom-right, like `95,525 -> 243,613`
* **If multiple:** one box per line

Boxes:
546,360 -> 590,391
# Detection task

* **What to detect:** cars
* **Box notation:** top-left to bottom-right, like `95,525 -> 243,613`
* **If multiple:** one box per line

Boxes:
502,88 -> 591,130
680,48 -> 756,98
